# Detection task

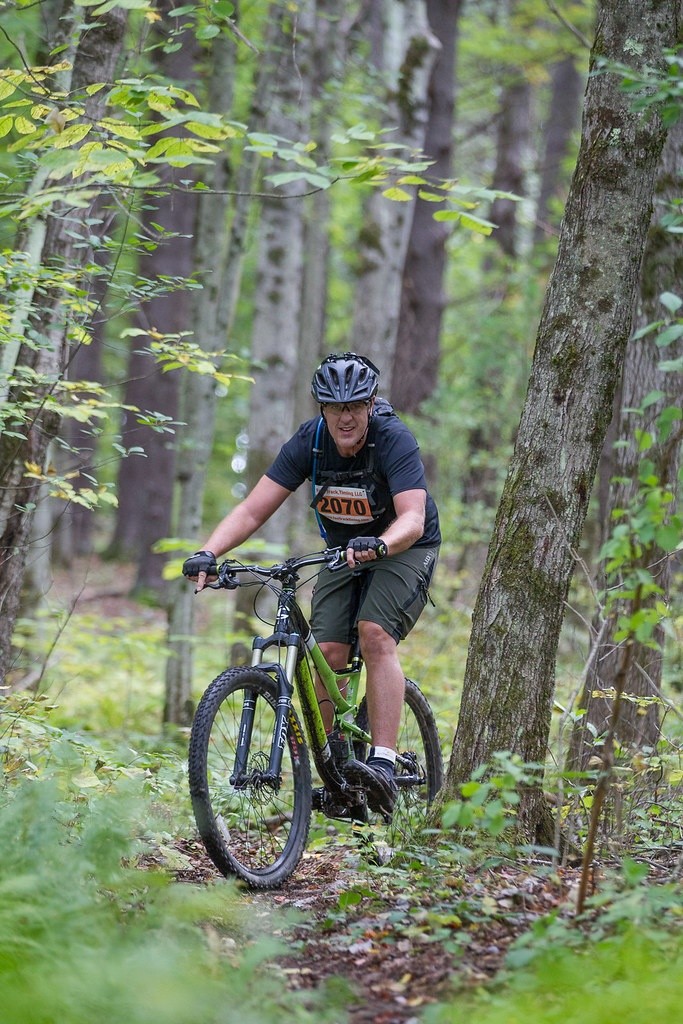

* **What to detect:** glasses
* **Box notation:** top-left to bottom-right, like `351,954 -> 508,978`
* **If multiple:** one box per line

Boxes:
321,401 -> 368,418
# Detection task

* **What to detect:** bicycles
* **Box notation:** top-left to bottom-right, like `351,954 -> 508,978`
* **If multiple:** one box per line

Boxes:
187,547 -> 444,890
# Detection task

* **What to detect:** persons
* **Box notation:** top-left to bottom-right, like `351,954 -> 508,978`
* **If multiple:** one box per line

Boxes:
181,351 -> 442,814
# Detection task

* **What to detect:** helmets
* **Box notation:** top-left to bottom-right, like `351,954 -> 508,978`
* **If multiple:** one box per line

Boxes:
310,352 -> 381,405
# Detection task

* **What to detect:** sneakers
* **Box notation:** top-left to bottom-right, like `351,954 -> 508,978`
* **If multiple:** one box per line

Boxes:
342,757 -> 398,812
311,786 -> 346,810
